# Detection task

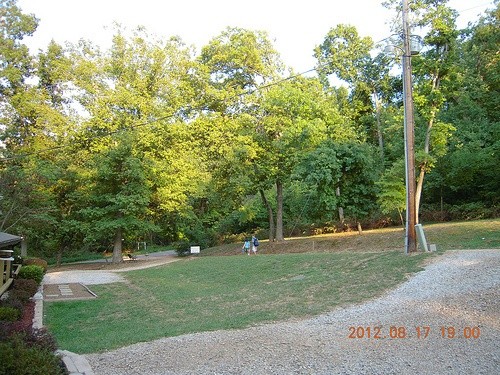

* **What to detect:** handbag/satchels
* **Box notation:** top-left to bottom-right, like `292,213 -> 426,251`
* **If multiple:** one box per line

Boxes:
241,249 -> 245,255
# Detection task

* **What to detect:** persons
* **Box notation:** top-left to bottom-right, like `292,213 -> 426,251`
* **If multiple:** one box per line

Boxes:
251,234 -> 258,255
242,239 -> 251,256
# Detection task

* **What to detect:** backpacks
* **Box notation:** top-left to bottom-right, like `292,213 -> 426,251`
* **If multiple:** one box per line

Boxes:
252,238 -> 258,246
